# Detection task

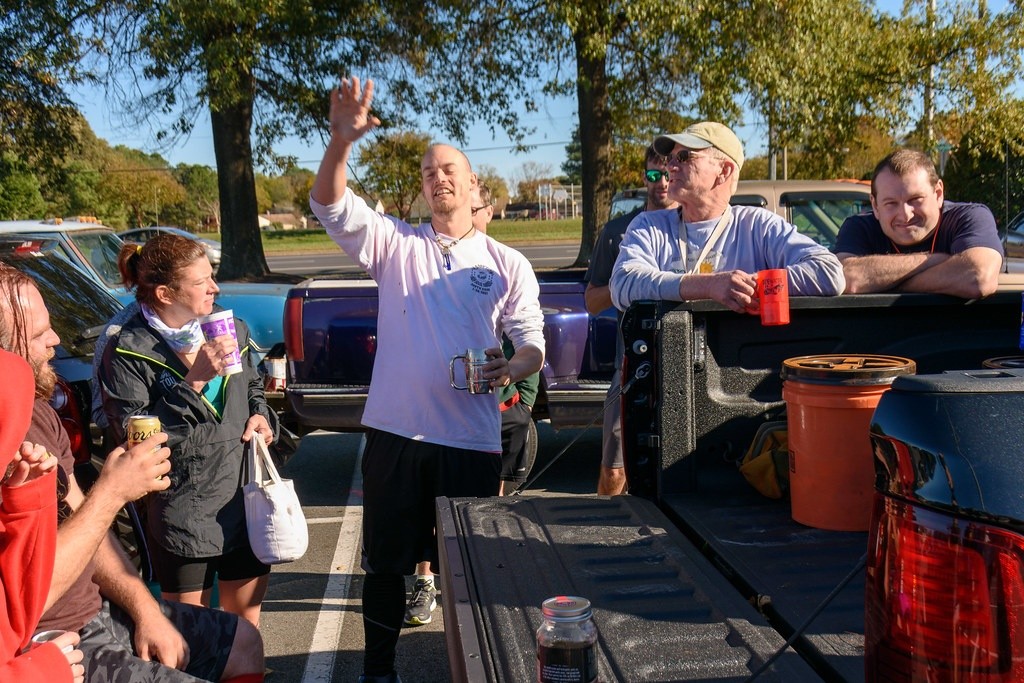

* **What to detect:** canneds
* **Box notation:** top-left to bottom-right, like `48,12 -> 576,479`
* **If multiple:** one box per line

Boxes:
128,414 -> 161,480
31,629 -> 74,667
536,596 -> 599,683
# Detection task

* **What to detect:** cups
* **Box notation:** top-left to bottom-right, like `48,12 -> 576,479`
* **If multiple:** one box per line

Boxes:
198,309 -> 243,377
449,347 -> 496,395
744,269 -> 790,326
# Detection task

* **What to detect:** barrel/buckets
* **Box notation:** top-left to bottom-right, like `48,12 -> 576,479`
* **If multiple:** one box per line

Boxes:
780,353 -> 917,531
263,356 -> 287,390
865,354 -> 1024,683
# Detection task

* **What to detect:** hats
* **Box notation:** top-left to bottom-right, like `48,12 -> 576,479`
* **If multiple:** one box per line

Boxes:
653,122 -> 744,169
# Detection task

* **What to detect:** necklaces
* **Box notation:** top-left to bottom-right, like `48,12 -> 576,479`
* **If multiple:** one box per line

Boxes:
431,223 -> 475,270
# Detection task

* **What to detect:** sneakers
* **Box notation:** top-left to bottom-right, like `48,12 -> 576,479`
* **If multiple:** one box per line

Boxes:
402,579 -> 438,625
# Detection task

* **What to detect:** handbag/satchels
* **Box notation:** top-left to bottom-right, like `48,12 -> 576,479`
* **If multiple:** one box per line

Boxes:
242,431 -> 309,564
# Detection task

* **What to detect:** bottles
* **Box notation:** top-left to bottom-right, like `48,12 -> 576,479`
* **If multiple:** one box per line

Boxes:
536,595 -> 599,683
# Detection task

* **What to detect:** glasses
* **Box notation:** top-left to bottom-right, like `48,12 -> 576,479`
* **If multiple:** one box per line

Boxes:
664,149 -> 726,164
645,169 -> 670,183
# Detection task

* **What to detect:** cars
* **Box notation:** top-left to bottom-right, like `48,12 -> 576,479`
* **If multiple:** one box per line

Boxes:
90,225 -> 221,285
0,211 -> 320,477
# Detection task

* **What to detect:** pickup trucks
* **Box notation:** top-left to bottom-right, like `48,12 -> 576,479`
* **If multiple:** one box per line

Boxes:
432,283 -> 1024,683
281,178 -> 874,492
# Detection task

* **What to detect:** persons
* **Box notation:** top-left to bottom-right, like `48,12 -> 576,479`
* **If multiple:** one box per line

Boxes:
586,122 -> 847,494
0,259 -> 265,683
407,182 -> 540,625
97,234 -> 277,627
311,80 -> 546,683
837,150 -> 1004,301
